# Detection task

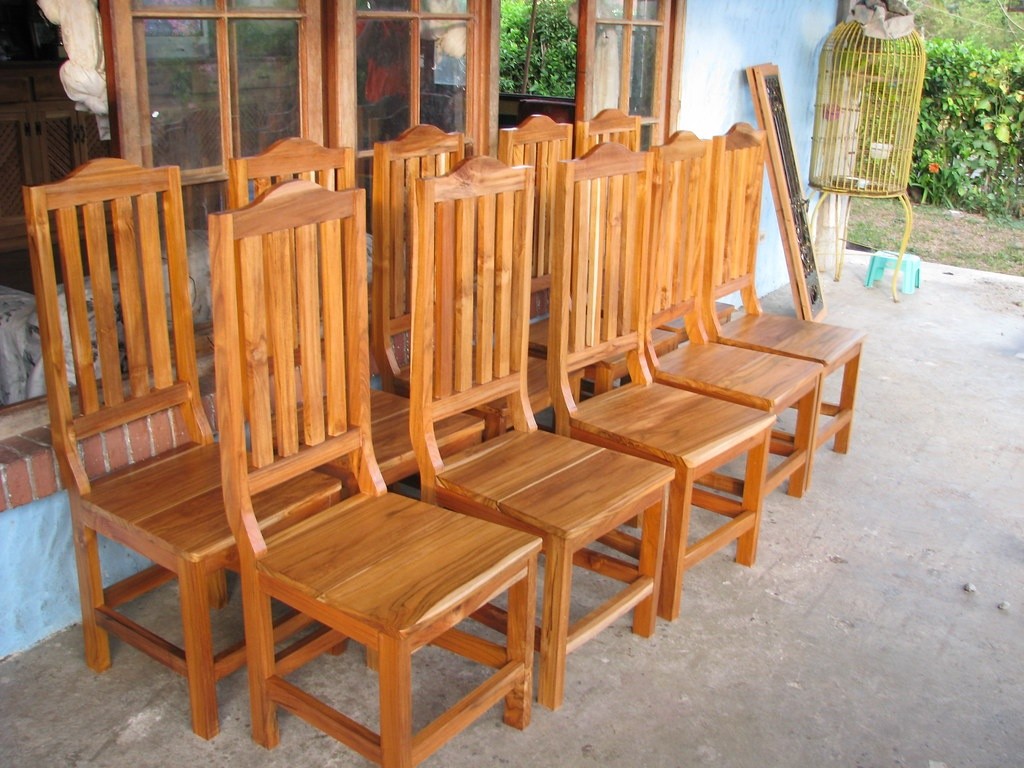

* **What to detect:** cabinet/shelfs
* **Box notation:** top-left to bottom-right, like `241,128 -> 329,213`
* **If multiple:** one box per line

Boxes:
0,59 -> 298,251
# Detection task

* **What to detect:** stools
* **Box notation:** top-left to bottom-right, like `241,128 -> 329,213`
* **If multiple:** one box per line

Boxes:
863,250 -> 922,293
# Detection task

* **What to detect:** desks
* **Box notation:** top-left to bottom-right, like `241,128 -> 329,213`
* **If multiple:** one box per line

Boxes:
803,175 -> 912,303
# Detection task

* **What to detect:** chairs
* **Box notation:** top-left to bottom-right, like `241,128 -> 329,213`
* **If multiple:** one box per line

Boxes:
369,92 -> 454,150
22,108 -> 867,768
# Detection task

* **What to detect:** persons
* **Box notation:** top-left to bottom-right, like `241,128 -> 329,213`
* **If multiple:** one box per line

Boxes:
356,0 -> 424,142
